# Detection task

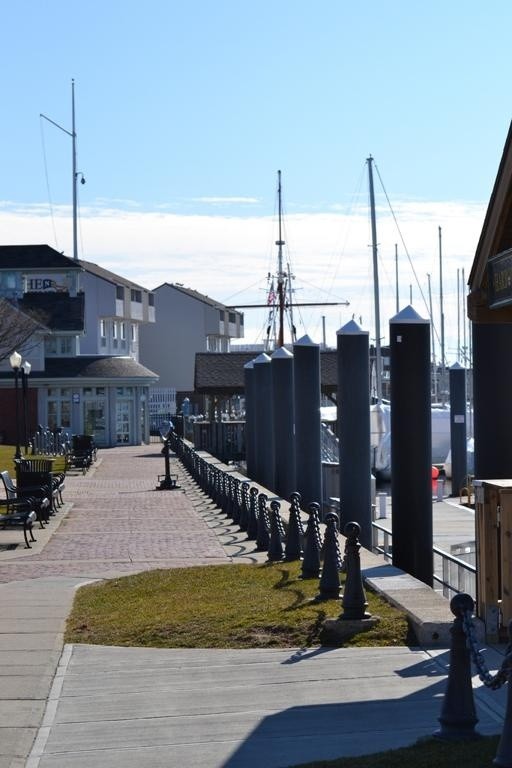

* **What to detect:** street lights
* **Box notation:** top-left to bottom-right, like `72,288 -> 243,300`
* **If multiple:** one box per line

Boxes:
8,350 -> 23,464
73,170 -> 85,261
20,360 -> 32,456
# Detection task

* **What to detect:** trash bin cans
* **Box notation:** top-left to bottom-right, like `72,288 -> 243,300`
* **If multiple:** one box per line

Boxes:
72,434 -> 95,466
14,458 -> 57,519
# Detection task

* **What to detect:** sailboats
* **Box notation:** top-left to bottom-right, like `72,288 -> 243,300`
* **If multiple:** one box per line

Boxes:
221,150 -> 475,482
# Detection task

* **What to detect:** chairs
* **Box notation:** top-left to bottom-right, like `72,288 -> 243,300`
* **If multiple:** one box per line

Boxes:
52,438 -> 98,516
0,470 -> 58,530
0,497 -> 36,549
